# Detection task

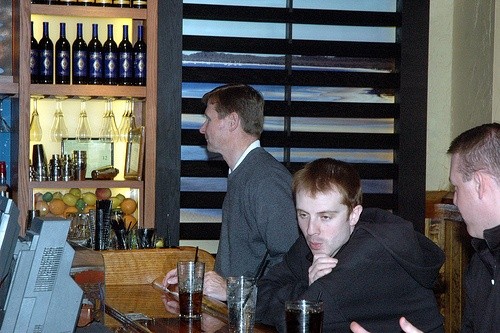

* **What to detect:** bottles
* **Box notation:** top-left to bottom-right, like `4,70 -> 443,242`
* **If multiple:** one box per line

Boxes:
0,161 -> 11,200
30,21 -> 147,87
31,0 -> 148,9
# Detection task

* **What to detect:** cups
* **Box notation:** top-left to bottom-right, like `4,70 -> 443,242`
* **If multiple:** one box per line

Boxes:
285,301 -> 324,333
177,261 -> 205,319
27,209 -> 40,230
66,210 -> 110,250
136,228 -> 156,249
29,144 -> 119,182
114,229 -> 132,250
226,276 -> 258,333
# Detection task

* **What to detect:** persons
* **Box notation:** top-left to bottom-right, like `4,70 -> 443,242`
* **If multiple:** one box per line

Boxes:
350,123 -> 500,333
162,84 -> 447,333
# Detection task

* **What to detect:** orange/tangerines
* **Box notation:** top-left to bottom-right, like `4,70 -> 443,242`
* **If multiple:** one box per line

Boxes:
34,193 -> 138,231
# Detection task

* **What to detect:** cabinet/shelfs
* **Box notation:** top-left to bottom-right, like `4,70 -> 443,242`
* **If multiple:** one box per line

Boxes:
13,0 -> 158,237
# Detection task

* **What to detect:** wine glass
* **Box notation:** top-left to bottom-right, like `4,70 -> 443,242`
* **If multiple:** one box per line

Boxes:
29,95 -> 143,144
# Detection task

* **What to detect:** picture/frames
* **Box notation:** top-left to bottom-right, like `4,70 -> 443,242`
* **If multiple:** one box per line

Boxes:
125,127 -> 145,180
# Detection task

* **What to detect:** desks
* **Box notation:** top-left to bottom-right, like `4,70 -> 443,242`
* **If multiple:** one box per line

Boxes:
102,283 -> 229,333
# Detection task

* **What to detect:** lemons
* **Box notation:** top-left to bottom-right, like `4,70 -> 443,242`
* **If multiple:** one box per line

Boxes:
59,188 -> 124,207
